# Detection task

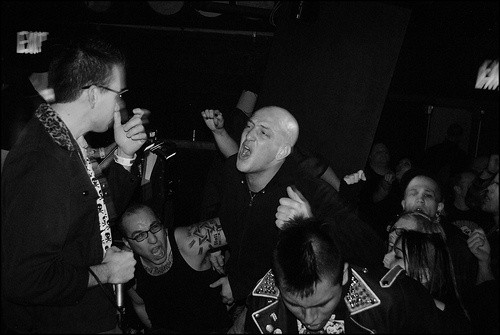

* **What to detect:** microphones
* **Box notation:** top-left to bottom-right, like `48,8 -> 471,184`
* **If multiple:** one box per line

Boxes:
113,239 -> 126,307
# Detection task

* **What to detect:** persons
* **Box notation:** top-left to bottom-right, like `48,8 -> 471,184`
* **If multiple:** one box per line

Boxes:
122,106 -> 331,335
0,36 -> 147,335
344,143 -> 500,335
244,217 -> 445,335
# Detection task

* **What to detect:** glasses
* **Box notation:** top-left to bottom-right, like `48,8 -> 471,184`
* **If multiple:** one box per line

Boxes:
386,225 -> 407,236
125,222 -> 164,242
81,82 -> 130,98
